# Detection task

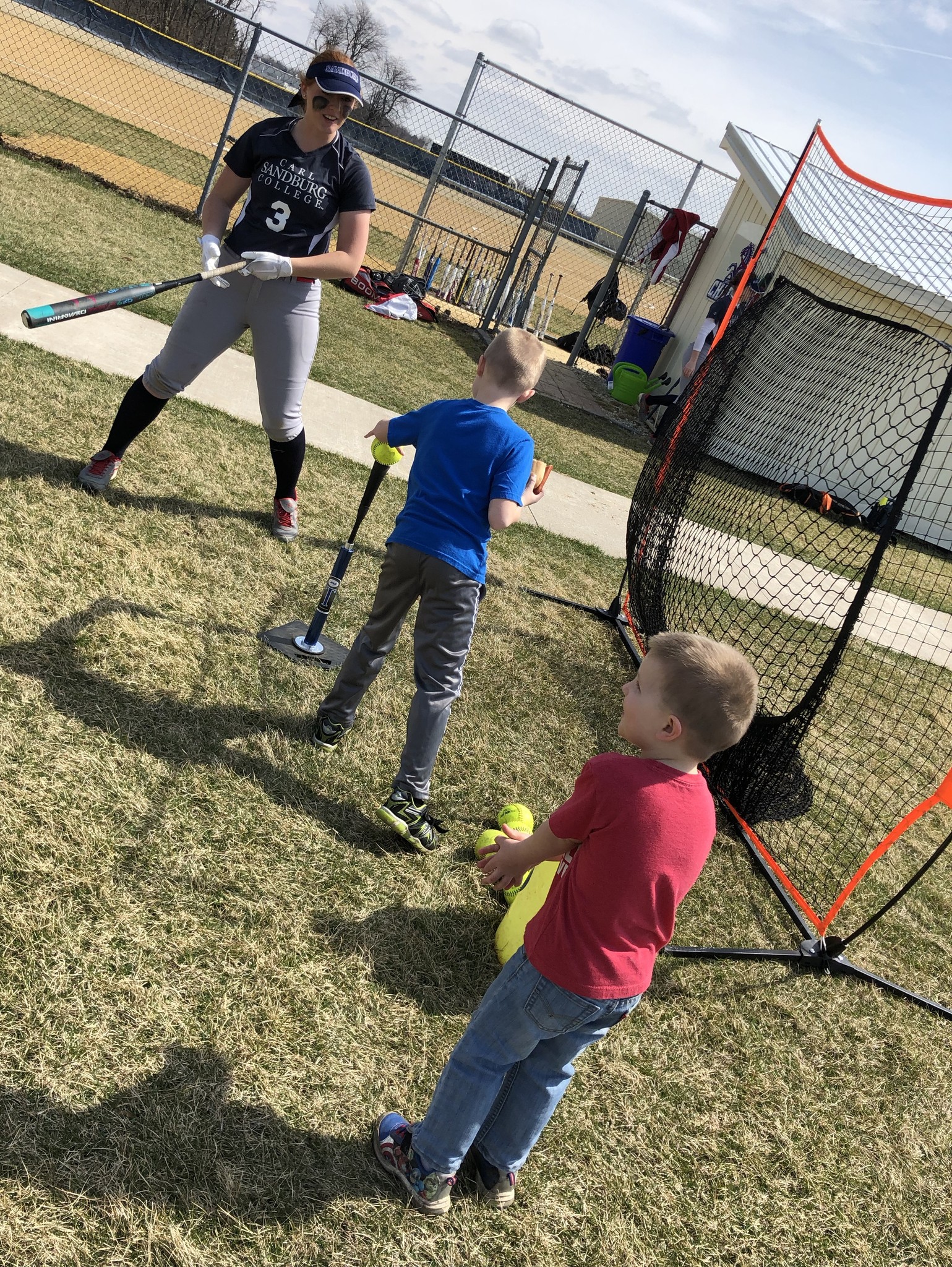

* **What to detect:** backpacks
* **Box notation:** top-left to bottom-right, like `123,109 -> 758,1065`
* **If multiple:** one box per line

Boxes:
582,272 -> 620,326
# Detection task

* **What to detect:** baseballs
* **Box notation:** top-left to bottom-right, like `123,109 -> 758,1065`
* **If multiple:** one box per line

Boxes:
502,868 -> 537,907
370,435 -> 404,467
496,802 -> 536,828
473,828 -> 511,862
500,820 -> 536,837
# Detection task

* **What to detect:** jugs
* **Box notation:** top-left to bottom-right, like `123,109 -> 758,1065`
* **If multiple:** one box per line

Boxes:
609,362 -> 672,405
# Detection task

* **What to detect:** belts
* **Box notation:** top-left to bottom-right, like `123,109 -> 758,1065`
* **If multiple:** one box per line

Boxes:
280,276 -> 314,283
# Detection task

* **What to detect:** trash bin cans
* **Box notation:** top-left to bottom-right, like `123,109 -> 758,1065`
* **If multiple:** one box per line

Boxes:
604,314 -> 677,386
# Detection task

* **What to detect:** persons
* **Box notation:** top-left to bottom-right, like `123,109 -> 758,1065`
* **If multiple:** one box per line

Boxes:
374,629 -> 759,1212
311,327 -> 548,853
638,269 -> 766,452
80,51 -> 376,541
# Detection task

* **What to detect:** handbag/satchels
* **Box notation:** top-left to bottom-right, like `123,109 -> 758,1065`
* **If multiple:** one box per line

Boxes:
391,273 -> 426,301
556,331 -> 590,359
588,344 -> 615,368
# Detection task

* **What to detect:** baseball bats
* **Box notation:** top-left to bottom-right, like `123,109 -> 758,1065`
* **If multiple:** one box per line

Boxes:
410,216 -> 563,342
20,256 -> 258,330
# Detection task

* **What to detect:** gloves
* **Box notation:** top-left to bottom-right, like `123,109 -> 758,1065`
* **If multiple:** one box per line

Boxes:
237,251 -> 293,281
198,234 -> 230,289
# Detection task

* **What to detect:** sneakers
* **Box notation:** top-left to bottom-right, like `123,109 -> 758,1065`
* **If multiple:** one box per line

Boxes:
272,488 -> 298,542
79,451 -> 121,491
374,1111 -> 456,1214
647,435 -> 667,451
468,1142 -> 517,1208
312,715 -> 353,753
376,785 -> 450,853
638,393 -> 650,421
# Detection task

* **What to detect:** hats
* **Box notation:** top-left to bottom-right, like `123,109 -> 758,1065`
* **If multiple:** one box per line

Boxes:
306,62 -> 364,108
734,277 -> 766,293
